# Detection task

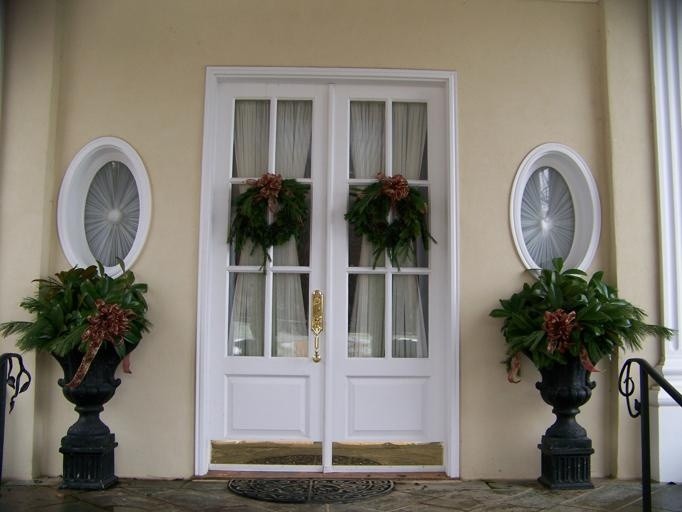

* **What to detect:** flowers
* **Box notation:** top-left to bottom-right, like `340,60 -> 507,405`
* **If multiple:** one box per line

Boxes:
343,170 -> 439,272
226,172 -> 312,258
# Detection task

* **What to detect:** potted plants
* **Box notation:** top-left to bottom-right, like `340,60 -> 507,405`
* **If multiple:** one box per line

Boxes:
486,261 -> 677,439
0,255 -> 155,435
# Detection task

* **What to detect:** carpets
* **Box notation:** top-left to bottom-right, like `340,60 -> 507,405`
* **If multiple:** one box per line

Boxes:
226,452 -> 395,504
